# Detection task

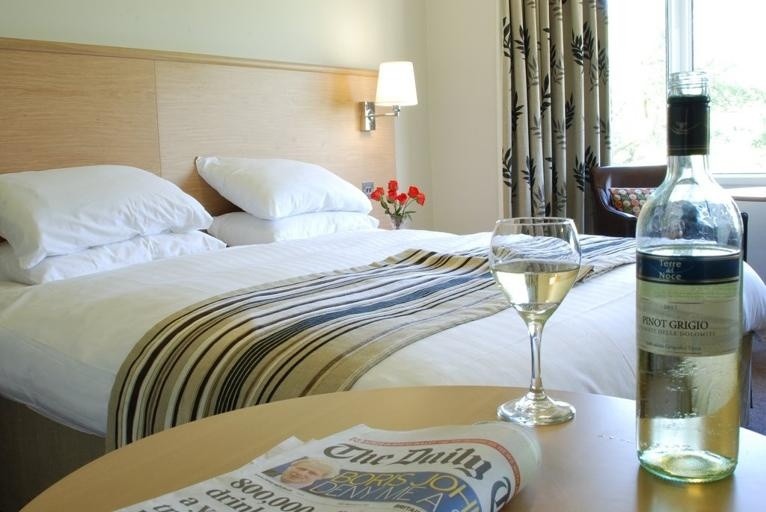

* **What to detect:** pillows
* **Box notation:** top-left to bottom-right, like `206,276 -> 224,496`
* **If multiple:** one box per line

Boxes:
0,231 -> 227,285
195,156 -> 373,220
0,164 -> 214,269
205,211 -> 381,248
608,187 -> 657,217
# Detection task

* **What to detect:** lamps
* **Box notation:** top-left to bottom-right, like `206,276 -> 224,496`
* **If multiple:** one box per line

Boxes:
358,61 -> 419,131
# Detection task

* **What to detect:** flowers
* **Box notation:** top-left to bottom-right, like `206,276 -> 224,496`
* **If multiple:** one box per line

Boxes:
371,181 -> 425,228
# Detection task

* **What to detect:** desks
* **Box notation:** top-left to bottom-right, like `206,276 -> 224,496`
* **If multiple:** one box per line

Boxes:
21,386 -> 766,512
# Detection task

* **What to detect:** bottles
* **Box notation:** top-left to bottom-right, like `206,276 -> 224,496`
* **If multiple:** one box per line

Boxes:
635,70 -> 745,483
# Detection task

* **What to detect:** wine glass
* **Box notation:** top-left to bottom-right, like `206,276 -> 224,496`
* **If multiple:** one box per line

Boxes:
488,216 -> 582,427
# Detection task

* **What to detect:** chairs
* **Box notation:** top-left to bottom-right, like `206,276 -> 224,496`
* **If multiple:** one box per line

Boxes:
590,166 -> 748,263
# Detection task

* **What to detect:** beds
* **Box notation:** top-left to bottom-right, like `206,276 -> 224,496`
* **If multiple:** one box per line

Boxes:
0,227 -> 753,511
